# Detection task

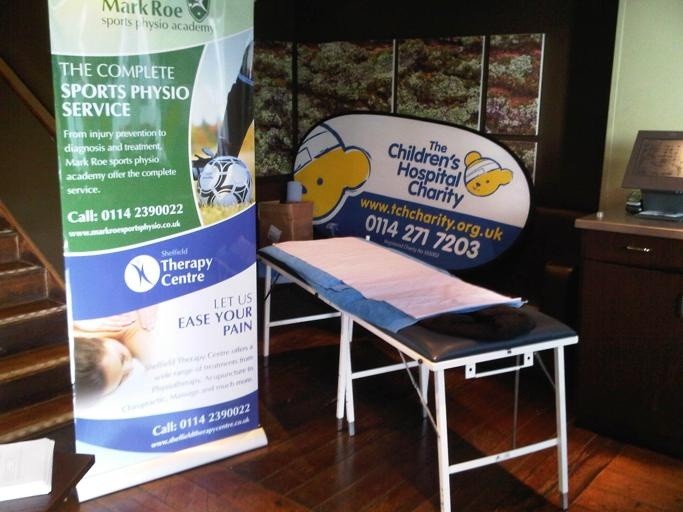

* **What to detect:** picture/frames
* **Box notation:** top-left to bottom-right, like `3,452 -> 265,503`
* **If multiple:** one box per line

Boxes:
621,129 -> 683,193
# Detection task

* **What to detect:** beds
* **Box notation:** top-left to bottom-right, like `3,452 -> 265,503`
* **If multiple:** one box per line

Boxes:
260,237 -> 579,512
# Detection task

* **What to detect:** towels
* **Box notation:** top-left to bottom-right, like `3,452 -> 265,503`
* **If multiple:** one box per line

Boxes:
0,437 -> 57,500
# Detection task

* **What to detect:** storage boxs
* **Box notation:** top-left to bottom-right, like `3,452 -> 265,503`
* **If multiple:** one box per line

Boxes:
257,197 -> 314,243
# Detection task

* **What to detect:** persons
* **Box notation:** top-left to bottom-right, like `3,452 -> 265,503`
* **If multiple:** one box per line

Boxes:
72,304 -> 163,401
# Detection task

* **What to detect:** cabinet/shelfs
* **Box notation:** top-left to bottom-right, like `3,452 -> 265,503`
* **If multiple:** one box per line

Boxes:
574,209 -> 683,460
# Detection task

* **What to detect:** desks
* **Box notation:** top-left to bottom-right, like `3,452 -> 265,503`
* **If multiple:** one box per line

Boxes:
0,450 -> 95,512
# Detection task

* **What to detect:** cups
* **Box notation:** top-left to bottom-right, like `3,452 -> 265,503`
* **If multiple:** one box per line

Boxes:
287,181 -> 302,204
